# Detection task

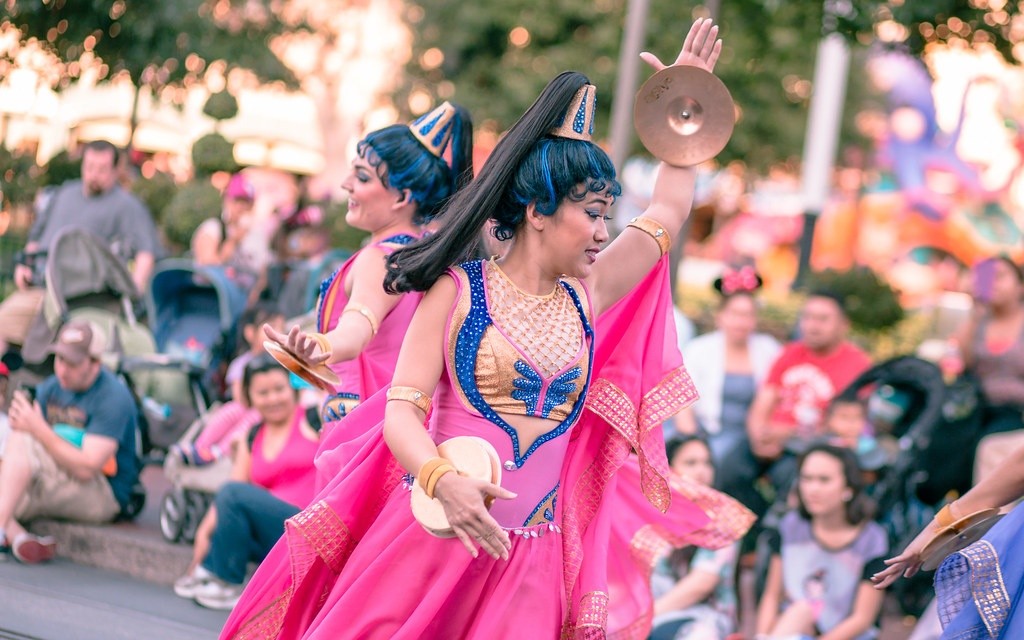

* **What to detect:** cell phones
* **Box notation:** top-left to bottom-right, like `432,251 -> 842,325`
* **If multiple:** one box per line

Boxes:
20,383 -> 38,404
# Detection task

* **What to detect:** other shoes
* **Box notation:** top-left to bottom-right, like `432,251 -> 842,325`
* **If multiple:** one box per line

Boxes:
0,539 -> 10,560
174,566 -> 214,598
13,533 -> 58,564
193,581 -> 245,610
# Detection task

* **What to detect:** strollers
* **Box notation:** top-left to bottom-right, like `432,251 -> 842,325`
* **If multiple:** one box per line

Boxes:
737,357 -> 957,590
158,403 -> 237,545
139,253 -> 265,406
39,228 -> 214,523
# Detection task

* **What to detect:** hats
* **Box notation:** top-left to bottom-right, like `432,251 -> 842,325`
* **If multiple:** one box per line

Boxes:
227,176 -> 252,204
46,318 -> 104,364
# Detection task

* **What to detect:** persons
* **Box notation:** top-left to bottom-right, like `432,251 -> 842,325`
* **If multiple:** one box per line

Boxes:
173,351 -> 334,610
756,443 -> 890,640
216,14 -> 724,639
1,317 -> 147,565
745,256 -> 1024,621
868,430 -> 1024,640
262,99 -> 758,640
642,434 -> 744,640
670,259 -> 786,634
0,137 -> 337,468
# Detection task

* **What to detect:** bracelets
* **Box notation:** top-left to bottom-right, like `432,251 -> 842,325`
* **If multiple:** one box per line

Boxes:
302,330 -> 332,365
417,458 -> 452,494
936,504 -> 956,529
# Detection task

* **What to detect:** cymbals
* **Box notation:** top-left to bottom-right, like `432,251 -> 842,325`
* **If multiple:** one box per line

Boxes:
409,435 -> 503,541
262,340 -> 339,397
633,65 -> 738,169
269,338 -> 342,387
